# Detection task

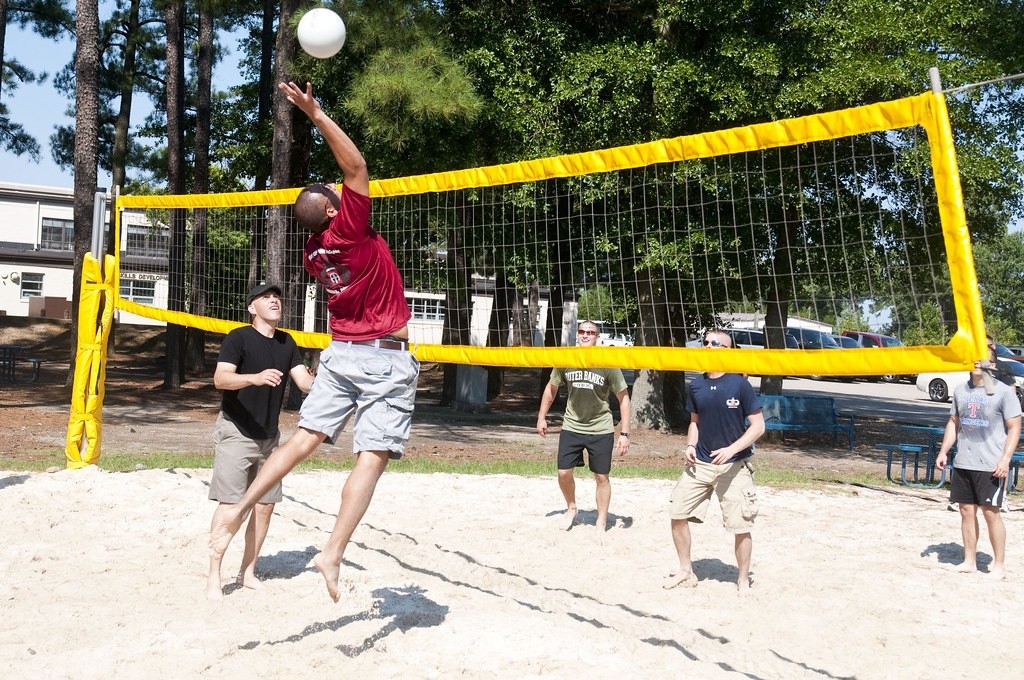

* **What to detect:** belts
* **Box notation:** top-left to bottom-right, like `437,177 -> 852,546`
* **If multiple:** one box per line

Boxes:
351,339 -> 410,351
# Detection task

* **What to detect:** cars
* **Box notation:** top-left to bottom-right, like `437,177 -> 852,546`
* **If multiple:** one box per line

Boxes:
685,325 -> 1024,416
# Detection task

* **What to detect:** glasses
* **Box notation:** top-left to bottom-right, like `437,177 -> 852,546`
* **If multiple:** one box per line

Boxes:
578,330 -> 597,335
703,340 -> 727,348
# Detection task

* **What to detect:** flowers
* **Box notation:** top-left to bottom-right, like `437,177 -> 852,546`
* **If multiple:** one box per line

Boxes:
896,425 -> 947,487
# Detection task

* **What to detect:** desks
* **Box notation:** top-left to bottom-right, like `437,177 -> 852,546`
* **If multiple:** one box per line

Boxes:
0,345 -> 32,387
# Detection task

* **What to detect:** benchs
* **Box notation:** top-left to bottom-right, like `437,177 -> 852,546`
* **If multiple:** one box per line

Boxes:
25,357 -> 48,384
741,396 -> 856,453
1007,451 -> 1024,494
874,444 -> 920,485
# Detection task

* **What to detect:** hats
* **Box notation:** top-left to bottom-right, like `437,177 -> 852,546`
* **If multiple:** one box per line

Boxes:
247,285 -> 281,306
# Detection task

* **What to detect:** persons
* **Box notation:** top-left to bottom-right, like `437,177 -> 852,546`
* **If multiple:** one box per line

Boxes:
664,329 -> 765,598
936,336 -> 1024,583
537,320 -> 631,546
207,80 -> 421,603
208,284 -> 316,599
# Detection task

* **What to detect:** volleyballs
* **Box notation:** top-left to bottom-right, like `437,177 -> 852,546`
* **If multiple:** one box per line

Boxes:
297,7 -> 346,59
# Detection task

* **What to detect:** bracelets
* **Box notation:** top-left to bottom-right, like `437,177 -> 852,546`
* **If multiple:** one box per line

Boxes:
687,445 -> 697,448
621,432 -> 630,436
